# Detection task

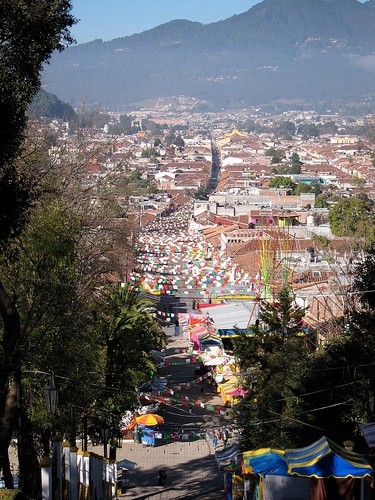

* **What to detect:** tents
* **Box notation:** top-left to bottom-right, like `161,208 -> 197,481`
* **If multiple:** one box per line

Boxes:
187,312 -> 256,407
215,436 -> 373,500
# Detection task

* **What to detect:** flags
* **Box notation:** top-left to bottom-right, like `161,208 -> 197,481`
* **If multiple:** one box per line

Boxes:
148,429 -> 230,441
156,310 -> 176,317
120,209 -> 255,297
149,379 -> 187,402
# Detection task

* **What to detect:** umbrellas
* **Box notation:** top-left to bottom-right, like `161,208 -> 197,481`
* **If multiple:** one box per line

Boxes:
137,414 -> 164,426
